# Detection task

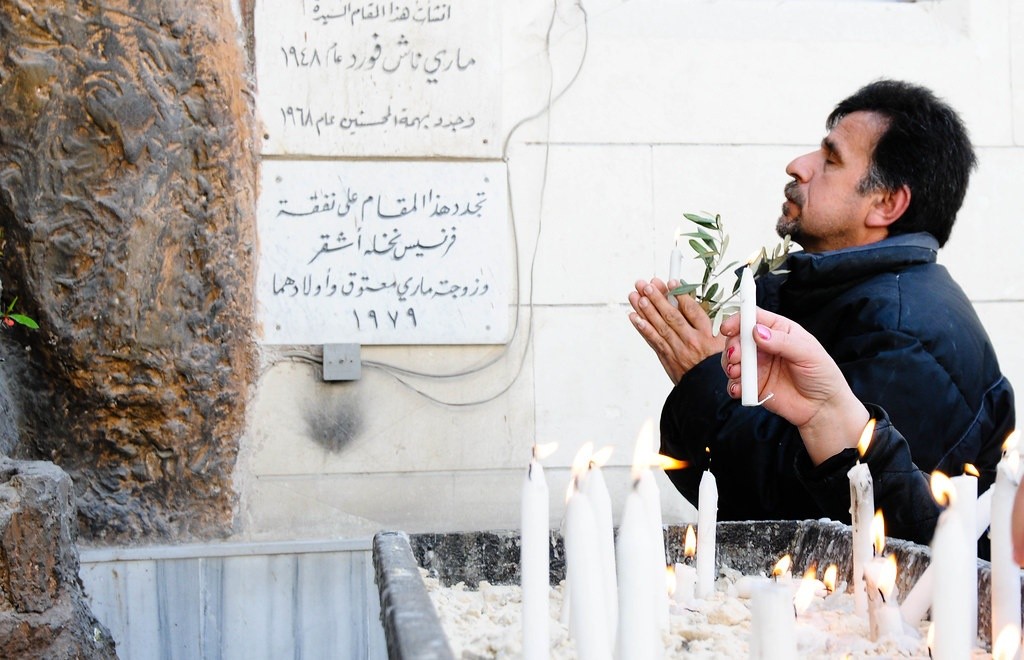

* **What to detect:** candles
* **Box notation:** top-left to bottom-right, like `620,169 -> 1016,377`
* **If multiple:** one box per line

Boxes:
755,555 -> 797,660
666,226 -> 684,308
630,453 -> 686,524
861,507 -> 899,642
900,483 -> 994,628
615,452 -> 661,660
949,466 -> 980,654
564,441 -> 611,660
874,553 -> 902,639
526,441 -> 560,507
521,460 -> 551,660
989,430 -> 1022,660
694,447 -> 718,601
739,250 -> 774,407
671,523 -> 697,603
845,418 -> 876,616
582,444 -> 618,639
929,470 -> 972,660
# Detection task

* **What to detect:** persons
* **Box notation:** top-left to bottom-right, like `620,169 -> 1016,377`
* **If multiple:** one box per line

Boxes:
720,306 -> 991,561
629,80 -> 1023,567
